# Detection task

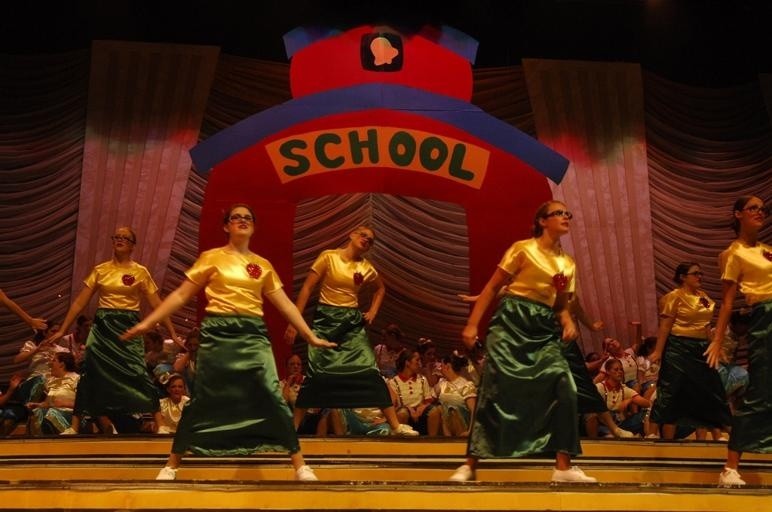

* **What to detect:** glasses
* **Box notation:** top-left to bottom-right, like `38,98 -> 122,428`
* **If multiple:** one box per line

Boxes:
356,232 -> 373,245
540,209 -> 572,219
111,235 -> 134,244
686,270 -> 702,276
227,214 -> 251,223
742,206 -> 767,214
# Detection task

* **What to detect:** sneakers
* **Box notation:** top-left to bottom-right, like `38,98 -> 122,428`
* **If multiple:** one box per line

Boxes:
391,424 -> 419,435
719,469 -> 746,486
156,467 -> 178,480
450,465 -> 476,481
295,465 -> 317,480
551,465 -> 597,482
59,428 -> 78,435
614,429 -> 633,438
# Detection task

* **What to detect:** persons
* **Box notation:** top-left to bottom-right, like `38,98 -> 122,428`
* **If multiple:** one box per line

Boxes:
449,200 -> 599,482
285,227 -> 420,438
0,287 -> 48,336
645,262 -> 733,441
700,195 -> 770,487
46,227 -> 189,435
457,284 -> 635,437
281,324 -> 485,437
712,327 -> 749,404
117,205 -> 336,482
1,314 -> 199,439
585,335 -> 662,439
